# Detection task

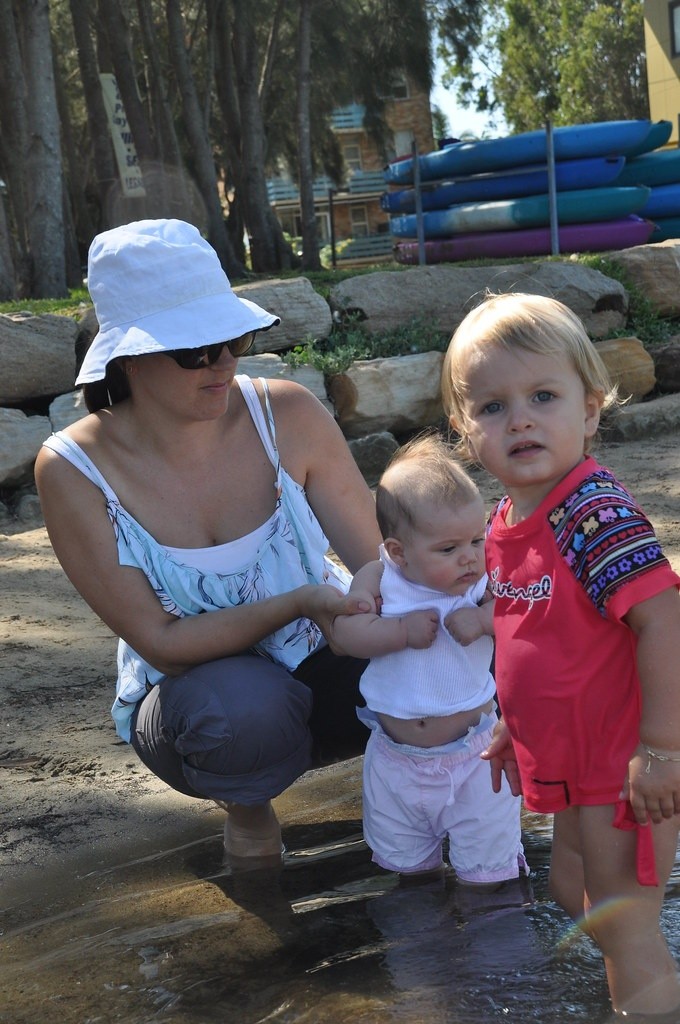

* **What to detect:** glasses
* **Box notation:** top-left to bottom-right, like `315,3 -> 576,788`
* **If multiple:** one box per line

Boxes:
159,329 -> 258,369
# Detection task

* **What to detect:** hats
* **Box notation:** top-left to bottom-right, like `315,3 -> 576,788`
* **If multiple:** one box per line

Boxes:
74,216 -> 283,385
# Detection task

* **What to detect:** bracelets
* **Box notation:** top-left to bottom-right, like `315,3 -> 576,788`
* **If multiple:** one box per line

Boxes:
639,740 -> 680,774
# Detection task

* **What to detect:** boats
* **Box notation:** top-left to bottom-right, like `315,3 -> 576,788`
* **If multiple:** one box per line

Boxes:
378,118 -> 680,267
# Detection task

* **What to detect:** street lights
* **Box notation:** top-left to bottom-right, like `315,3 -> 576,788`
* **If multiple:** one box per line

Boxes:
328,188 -> 351,271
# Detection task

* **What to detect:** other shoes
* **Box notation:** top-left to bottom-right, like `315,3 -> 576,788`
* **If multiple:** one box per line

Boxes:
224,802 -> 286,892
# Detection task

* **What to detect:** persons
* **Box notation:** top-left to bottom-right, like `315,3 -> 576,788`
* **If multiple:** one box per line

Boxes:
330,432 -> 530,884
34,219 -> 384,856
440,293 -> 680,1015
